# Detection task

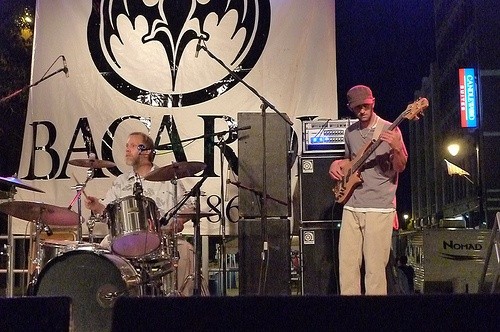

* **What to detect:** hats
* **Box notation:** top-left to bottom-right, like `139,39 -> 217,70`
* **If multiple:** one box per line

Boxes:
346,85 -> 375,109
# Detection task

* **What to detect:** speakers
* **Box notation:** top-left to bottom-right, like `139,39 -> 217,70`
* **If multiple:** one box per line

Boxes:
237,111 -> 293,217
299,227 -> 343,297
299,155 -> 344,223
237,220 -> 292,296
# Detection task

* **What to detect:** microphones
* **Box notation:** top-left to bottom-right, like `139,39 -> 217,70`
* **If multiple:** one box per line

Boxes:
42,223 -> 53,237
63,57 -> 69,78
137,144 -> 145,154
196,38 -> 201,58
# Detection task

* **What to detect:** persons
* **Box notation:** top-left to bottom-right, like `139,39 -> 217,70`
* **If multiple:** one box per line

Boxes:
329,85 -> 408,296
84,132 -> 196,297
